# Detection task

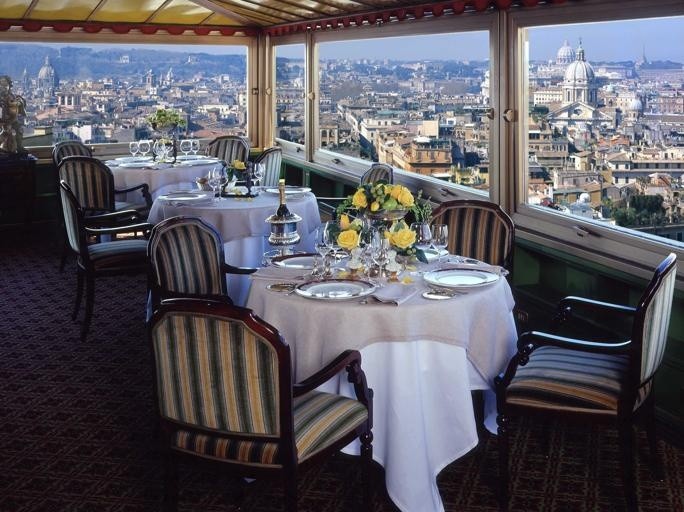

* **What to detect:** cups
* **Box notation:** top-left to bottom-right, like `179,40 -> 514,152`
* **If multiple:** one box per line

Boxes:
410,222 -> 432,250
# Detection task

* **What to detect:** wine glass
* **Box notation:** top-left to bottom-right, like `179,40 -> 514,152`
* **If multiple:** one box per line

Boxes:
253,164 -> 267,194
180,140 -> 191,161
323,220 -> 346,276
315,228 -> 333,279
191,140 -> 200,161
140,140 -> 149,161
208,171 -> 216,202
431,224 -> 448,264
128,143 -> 139,163
372,238 -> 390,289
358,229 -> 376,283
212,167 -> 227,203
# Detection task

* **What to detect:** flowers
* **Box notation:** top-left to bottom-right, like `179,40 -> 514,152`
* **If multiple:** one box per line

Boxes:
334,184 -> 433,285
144,109 -> 185,125
227,160 -> 246,181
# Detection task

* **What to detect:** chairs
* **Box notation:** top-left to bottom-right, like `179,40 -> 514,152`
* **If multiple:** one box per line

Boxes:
493,253 -> 676,512
432,199 -> 529,334
332,164 -> 393,221
233,147 -> 282,187
58,157 -> 153,276
52,141 -> 92,166
205,136 -> 248,164
148,300 -> 374,512
59,181 -> 153,342
148,216 -> 258,312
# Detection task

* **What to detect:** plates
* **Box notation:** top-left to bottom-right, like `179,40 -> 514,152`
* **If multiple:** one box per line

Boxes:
183,161 -> 219,166
116,158 -> 154,163
265,186 -> 311,195
270,254 -> 338,273
158,196 -> 206,202
117,163 -> 149,170
295,278 -> 375,302
423,269 -> 504,291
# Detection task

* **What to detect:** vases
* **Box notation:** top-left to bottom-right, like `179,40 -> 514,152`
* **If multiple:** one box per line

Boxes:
363,208 -> 408,278
153,125 -> 174,162
224,173 -> 237,190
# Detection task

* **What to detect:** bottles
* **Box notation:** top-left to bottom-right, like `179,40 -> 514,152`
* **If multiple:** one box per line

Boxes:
276,182 -> 291,219
